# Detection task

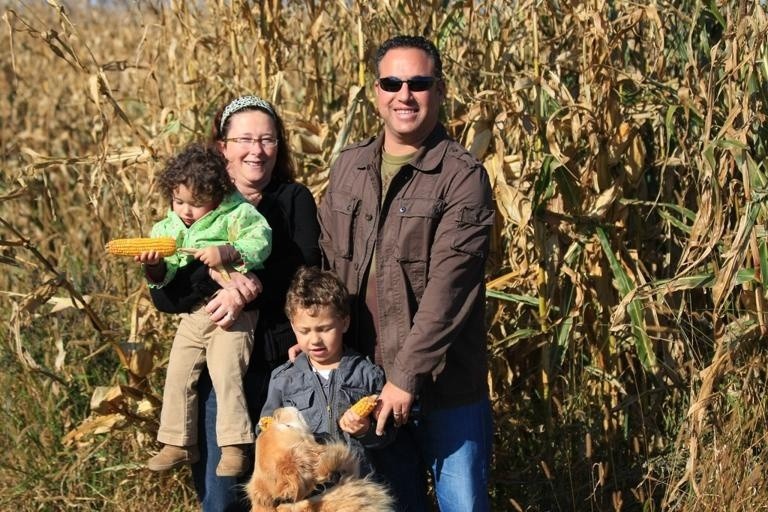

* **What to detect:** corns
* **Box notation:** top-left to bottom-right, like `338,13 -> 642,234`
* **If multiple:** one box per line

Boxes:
103,236 -> 177,257
130,336 -> 157,378
257,415 -> 274,433
350,394 -> 379,419
466,112 -> 492,161
607,118 -> 646,186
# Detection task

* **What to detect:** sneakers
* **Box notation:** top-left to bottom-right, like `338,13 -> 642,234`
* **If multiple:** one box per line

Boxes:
216,445 -> 250,476
148,445 -> 199,471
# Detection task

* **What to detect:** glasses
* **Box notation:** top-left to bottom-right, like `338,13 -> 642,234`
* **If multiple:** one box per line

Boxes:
379,78 -> 441,91
224,138 -> 279,147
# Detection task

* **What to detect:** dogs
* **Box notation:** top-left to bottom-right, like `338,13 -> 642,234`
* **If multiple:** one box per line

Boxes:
242,405 -> 400,512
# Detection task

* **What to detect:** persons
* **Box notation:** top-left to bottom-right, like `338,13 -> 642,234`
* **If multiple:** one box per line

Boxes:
256,265 -> 408,482
150,95 -> 321,512
133,144 -> 272,477
317,35 -> 498,512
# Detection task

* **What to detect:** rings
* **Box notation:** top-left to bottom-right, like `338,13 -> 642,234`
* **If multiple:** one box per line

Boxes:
393,413 -> 401,416
228,312 -> 235,320
